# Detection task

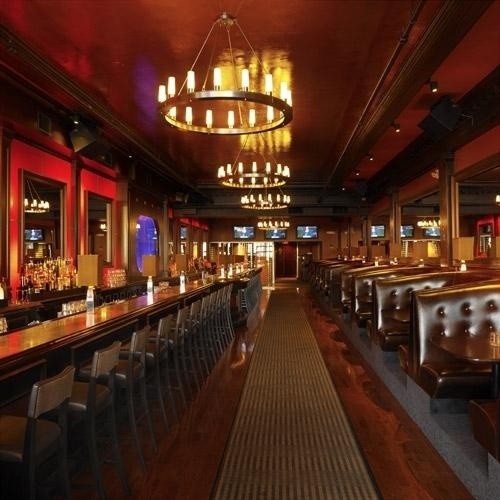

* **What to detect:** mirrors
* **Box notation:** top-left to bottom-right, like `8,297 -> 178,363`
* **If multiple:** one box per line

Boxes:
176,221 -> 210,263
85,191 -> 113,263
20,169 -> 66,263
395,185 -> 440,265
340,207 -> 390,261
450,151 -> 500,269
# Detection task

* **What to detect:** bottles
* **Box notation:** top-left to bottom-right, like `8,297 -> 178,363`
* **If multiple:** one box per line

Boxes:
86,286 -> 95,314
179,270 -> 186,288
146,275 -> 154,295
103,267 -> 127,289
337,253 -> 468,272
0,277 -> 7,300
220,262 -> 244,277
27,258 -> 77,296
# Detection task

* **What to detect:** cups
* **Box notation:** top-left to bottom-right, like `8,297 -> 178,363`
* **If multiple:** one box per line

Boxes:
0,317 -> 8,333
59,299 -> 85,317
489,332 -> 500,347
202,271 -> 208,279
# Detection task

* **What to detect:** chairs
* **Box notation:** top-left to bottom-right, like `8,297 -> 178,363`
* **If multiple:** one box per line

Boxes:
0,268 -> 262,500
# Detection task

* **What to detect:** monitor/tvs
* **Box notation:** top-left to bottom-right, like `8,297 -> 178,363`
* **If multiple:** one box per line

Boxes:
265,230 -> 287,239
24,227 -> 44,241
424,229 -> 441,237
232,224 -> 256,239
296,225 -> 318,239
371,224 -> 385,237
401,224 -> 414,237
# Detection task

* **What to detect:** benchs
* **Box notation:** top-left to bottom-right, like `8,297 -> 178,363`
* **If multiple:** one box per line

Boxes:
311,256 -> 500,499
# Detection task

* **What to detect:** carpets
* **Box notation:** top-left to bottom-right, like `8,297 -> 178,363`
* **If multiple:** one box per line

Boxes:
222,283 -> 382,499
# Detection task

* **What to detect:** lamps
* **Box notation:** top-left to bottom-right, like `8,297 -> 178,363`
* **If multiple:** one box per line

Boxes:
23,178 -> 49,213
256,214 -> 291,231
156,12 -> 292,135
237,188 -> 289,212
417,202 -> 441,229
215,136 -> 292,186
